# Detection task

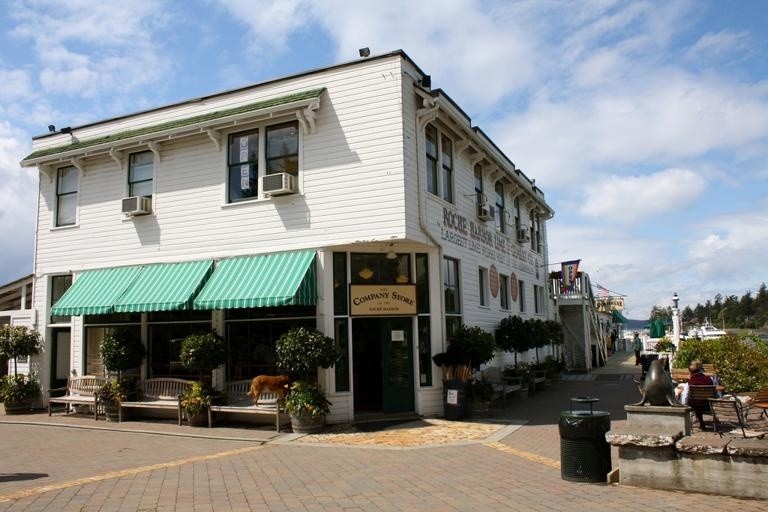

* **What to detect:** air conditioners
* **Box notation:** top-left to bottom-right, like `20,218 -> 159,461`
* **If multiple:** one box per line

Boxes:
122,196 -> 152,216
262,172 -> 296,196
517,229 -> 529,243
478,202 -> 495,221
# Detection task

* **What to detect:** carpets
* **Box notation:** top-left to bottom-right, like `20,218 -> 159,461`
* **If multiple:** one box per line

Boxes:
351,417 -> 438,432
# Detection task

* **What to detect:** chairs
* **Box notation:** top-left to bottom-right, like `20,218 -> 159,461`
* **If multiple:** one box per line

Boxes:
687,385 -> 768,440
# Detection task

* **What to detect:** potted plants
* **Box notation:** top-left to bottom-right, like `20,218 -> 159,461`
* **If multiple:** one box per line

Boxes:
0,324 -> 44,416
177,326 -> 228,428
94,327 -> 148,421
276,326 -> 346,434
441,315 -> 567,420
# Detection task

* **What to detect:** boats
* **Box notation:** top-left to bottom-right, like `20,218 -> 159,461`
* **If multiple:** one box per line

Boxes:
687,315 -> 728,342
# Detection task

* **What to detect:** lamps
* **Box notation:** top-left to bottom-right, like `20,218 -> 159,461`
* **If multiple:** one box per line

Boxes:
396,264 -> 408,283
385,243 -> 397,259
358,255 -> 373,279
61,127 -> 79,144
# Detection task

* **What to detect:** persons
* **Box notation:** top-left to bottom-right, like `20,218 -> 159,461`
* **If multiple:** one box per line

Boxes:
680,361 -> 719,409
633,332 -> 642,365
609,325 -> 618,353
673,356 -> 706,399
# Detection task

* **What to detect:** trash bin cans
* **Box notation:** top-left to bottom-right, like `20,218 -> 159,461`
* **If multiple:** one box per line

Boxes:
560,396 -> 611,483
641,349 -> 658,380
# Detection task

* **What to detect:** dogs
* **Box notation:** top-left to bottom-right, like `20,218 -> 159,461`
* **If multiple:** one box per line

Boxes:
246,373 -> 292,407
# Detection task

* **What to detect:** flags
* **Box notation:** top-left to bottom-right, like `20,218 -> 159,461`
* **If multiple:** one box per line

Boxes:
561,261 -> 579,290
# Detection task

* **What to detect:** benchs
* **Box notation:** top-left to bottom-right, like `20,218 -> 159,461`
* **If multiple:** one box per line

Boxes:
671,363 -> 715,383
206,378 -> 290,434
46,376 -> 112,421
117,378 -> 194,427
